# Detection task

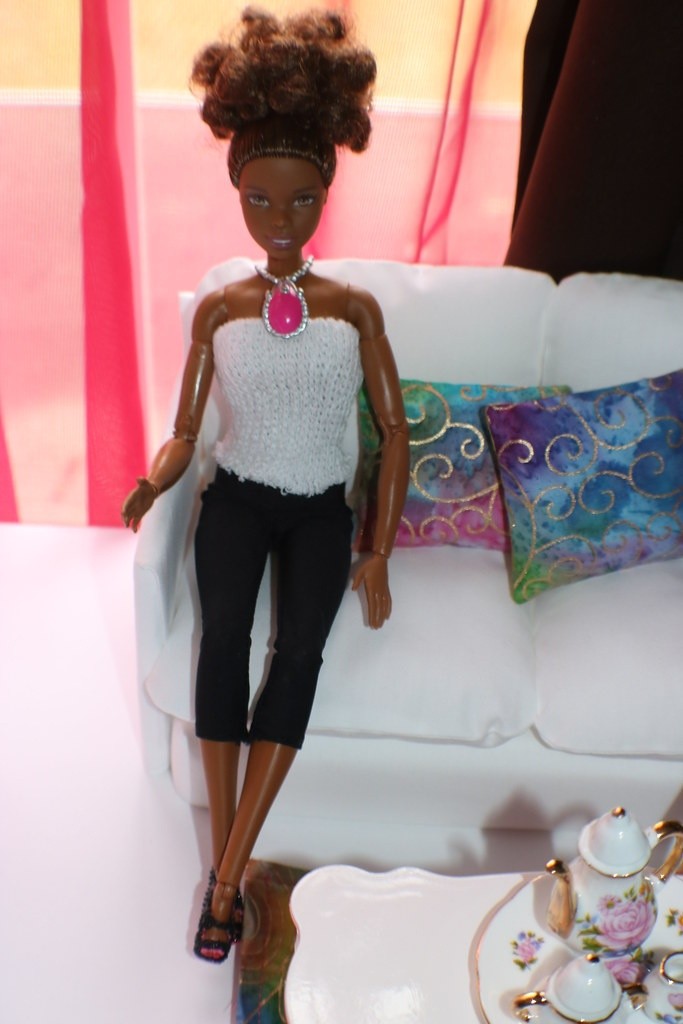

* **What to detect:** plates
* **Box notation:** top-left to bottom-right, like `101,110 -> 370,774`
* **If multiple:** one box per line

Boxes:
474,871 -> 682,1023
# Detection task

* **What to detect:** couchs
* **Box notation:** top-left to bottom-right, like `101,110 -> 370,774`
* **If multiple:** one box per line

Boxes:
129,256 -> 683,875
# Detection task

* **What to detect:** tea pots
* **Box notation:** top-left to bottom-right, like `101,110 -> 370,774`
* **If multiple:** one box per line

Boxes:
544,805 -> 683,961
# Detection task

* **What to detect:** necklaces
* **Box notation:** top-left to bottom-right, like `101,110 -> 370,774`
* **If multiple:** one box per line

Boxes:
253,254 -> 316,339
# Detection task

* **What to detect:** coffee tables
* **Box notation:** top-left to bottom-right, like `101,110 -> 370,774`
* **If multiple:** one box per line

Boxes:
281,860 -> 683,1023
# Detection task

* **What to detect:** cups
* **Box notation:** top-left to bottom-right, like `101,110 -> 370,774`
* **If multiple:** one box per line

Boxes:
509,952 -> 635,1024
623,949 -> 683,1024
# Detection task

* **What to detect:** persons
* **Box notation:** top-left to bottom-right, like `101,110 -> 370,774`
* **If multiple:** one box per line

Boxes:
125,7 -> 408,962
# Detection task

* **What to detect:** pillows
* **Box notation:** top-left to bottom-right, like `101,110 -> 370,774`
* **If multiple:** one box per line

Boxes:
480,366 -> 682,609
348,375 -> 574,553
